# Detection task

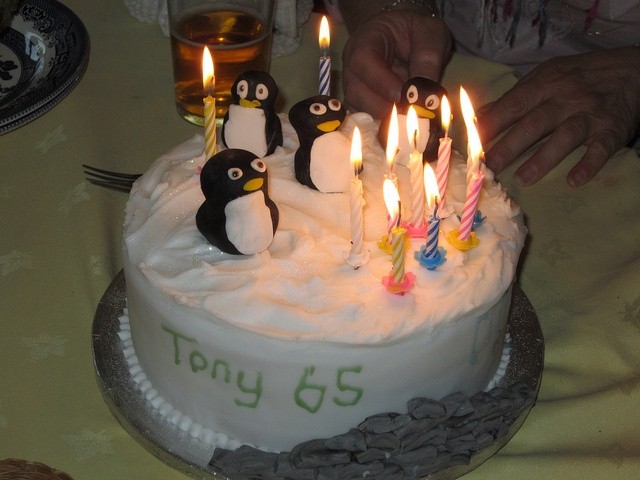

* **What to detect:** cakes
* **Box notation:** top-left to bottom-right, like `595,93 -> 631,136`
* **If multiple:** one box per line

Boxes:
119,54 -> 529,480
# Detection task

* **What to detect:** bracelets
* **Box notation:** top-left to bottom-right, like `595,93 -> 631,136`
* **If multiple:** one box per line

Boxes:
380,0 -> 436,18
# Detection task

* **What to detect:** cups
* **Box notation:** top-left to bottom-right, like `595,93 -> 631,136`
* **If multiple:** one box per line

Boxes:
167,0 -> 277,127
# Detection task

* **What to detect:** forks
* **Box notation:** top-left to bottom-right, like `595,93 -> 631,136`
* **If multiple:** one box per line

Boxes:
82,164 -> 144,194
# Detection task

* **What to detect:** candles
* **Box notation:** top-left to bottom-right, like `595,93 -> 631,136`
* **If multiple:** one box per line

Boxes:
421,161 -> 443,260
383,103 -> 403,244
405,104 -> 425,230
349,125 -> 367,255
434,94 -> 453,211
381,178 -> 409,284
460,86 -> 482,212
456,120 -> 486,241
199,46 -> 218,161
317,13 -> 333,99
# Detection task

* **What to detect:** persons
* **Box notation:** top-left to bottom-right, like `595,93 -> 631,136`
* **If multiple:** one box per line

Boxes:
340,1 -> 638,188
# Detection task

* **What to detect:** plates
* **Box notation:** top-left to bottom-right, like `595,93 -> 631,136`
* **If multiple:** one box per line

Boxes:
0,0 -> 91,136
